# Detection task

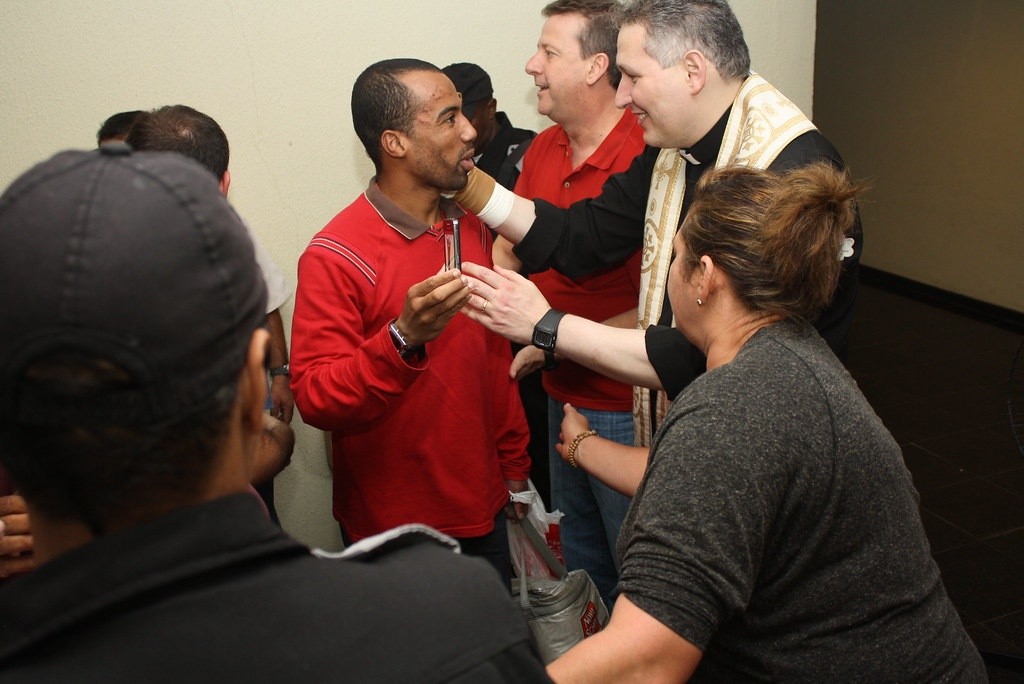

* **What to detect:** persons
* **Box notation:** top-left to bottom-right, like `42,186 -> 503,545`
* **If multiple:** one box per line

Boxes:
491,0 -> 648,614
0,103 -> 300,577
544,164 -> 993,684
441,61 -> 539,194
0,144 -> 554,684
445,0 -> 864,446
293,58 -> 530,584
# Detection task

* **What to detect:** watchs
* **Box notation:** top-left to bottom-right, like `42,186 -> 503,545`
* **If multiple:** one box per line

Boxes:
532,308 -> 565,352
270,362 -> 290,376
389,324 -> 422,362
543,350 -> 558,371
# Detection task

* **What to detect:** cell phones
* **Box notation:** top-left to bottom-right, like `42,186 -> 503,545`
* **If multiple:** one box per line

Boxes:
443,218 -> 461,273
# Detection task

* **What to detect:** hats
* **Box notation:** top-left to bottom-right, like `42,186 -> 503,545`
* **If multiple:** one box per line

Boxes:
0,142 -> 295,394
441,62 -> 493,105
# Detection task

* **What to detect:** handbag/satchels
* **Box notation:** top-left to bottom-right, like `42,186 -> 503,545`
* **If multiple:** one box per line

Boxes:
505,477 -> 569,583
499,512 -> 611,667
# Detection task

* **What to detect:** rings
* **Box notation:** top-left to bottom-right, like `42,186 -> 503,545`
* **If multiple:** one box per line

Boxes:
482,300 -> 488,312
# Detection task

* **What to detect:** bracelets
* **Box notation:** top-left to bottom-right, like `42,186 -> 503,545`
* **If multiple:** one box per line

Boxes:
568,430 -> 598,468
